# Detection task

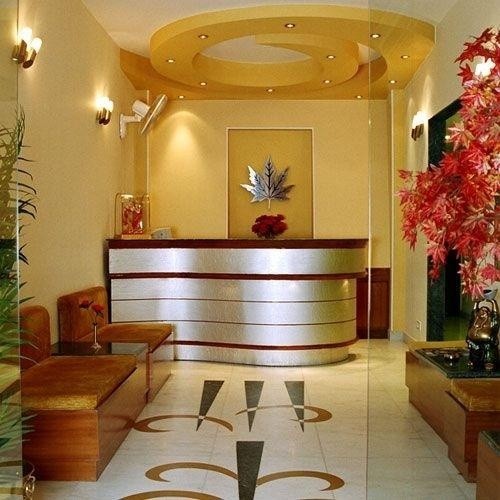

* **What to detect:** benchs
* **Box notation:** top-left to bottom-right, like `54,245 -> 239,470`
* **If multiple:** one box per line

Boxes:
19,286 -> 174,484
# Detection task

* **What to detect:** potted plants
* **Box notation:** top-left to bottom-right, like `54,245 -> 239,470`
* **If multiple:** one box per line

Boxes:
1,103 -> 40,500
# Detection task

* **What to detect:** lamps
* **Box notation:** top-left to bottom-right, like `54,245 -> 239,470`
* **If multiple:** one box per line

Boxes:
11,26 -> 43,69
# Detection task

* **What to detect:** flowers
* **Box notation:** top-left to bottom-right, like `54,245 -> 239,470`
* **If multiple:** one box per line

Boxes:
251,212 -> 288,240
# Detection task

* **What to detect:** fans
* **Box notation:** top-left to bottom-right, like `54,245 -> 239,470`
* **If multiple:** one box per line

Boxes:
119,94 -> 168,140
